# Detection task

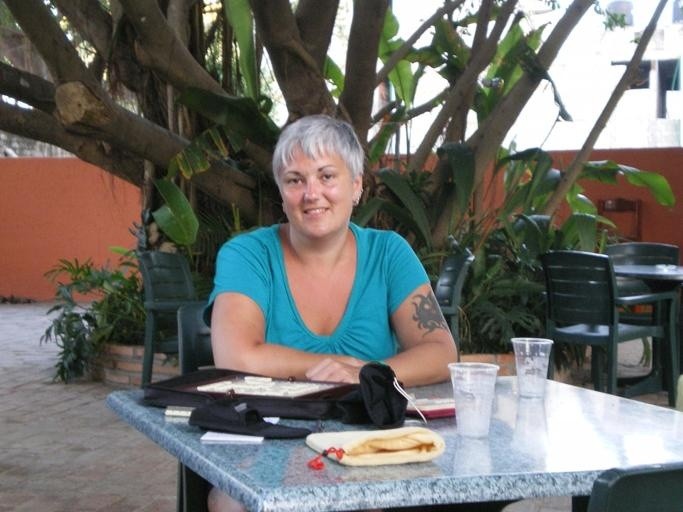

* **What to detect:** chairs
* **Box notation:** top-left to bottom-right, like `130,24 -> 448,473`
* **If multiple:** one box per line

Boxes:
536,248 -> 679,410
132,242 -> 205,389
599,242 -> 679,327
434,246 -> 474,365
170,298 -> 252,512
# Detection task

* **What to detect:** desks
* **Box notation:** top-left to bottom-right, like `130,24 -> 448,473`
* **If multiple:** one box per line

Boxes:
612,263 -> 683,400
106,371 -> 683,511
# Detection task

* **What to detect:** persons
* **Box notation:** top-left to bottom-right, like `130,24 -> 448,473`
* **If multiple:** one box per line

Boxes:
202,113 -> 573,512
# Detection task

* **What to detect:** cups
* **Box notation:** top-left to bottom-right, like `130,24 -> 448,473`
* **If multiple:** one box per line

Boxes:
453,439 -> 492,475
511,337 -> 554,400
512,399 -> 549,455
449,362 -> 500,439
